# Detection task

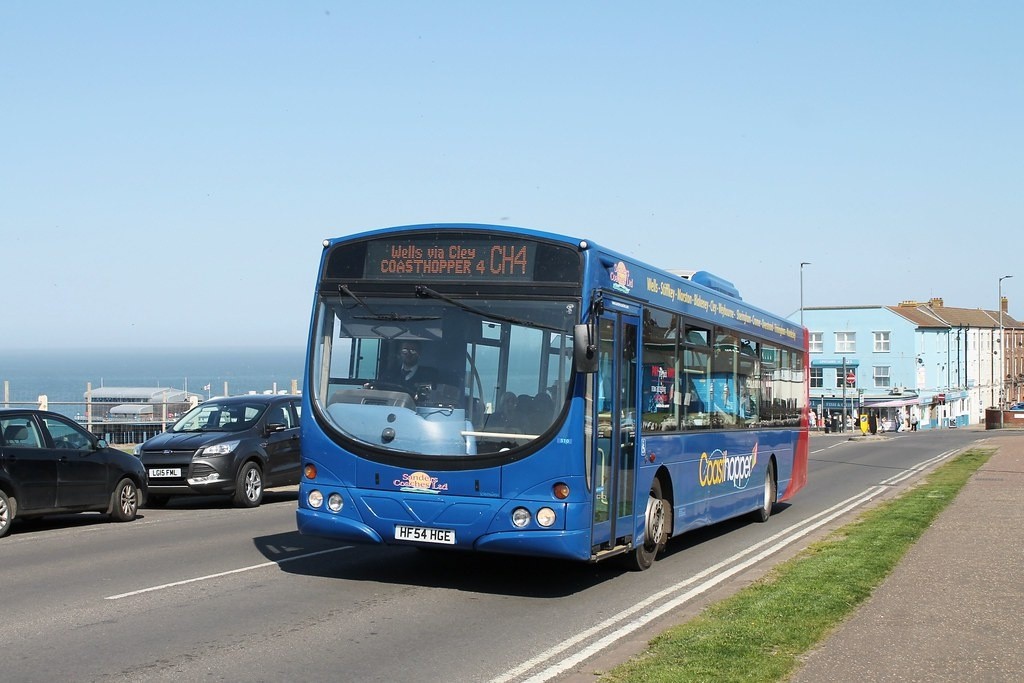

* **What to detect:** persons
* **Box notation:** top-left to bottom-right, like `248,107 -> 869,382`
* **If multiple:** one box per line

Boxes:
363,341 -> 435,399
499,380 -> 569,432
808,409 -> 917,433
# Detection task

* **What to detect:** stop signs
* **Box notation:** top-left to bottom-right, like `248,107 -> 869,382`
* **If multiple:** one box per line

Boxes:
938,394 -> 945,401
847,374 -> 855,384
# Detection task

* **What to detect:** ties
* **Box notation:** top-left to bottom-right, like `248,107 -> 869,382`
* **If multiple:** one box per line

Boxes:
402,368 -> 412,380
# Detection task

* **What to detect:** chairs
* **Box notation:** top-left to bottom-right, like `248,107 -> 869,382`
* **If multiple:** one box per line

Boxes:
281,409 -> 288,428
494,392 -> 551,432
4,425 -> 29,447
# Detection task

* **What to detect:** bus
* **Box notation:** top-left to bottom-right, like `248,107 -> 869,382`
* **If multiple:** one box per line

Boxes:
299,223 -> 811,575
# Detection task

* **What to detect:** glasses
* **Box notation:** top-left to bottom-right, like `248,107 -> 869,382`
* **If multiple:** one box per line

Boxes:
401,348 -> 419,355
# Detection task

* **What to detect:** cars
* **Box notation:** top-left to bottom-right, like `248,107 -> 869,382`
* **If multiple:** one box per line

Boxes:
139,395 -> 303,508
0,409 -> 150,539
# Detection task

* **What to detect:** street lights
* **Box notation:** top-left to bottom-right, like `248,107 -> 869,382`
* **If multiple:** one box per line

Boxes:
998,275 -> 1014,429
797,261 -> 811,328
